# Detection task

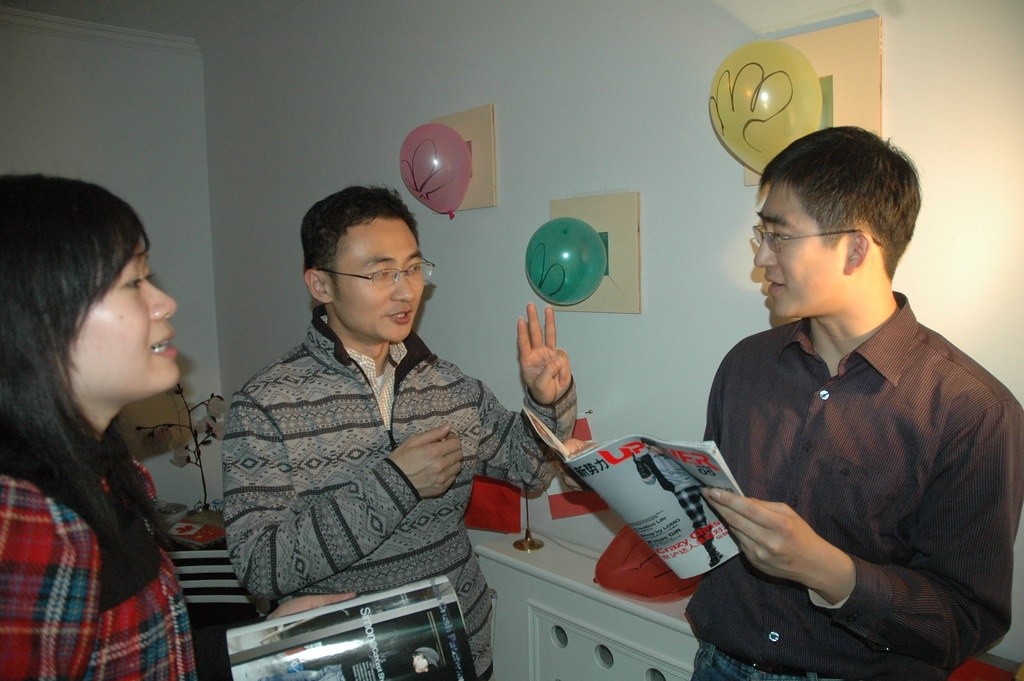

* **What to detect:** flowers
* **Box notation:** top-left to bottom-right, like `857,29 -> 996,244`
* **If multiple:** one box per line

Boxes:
136,381 -> 225,506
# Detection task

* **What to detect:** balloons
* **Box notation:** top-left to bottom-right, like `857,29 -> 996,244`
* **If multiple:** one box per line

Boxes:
709,41 -> 823,175
400,123 -> 472,219
592,526 -> 697,603
525,217 -> 607,305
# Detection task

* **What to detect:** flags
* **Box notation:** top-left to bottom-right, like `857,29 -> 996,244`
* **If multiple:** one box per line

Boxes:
544,413 -> 610,518
466,475 -> 522,533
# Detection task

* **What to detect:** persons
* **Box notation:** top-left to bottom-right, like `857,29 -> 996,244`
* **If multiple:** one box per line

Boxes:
564,124 -> 1023,681
0,173 -> 355,681
223,185 -> 578,681
632,438 -> 743,567
260,647 -> 439,681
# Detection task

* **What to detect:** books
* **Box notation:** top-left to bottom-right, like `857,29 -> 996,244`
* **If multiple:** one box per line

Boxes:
521,404 -> 745,579
167,520 -> 224,545
225,575 -> 477,681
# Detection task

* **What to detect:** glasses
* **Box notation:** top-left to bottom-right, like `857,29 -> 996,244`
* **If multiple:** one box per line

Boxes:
752,225 -> 883,254
317,258 -> 435,290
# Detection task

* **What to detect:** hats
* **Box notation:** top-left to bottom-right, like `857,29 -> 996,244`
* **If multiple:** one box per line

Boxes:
414,647 -> 439,667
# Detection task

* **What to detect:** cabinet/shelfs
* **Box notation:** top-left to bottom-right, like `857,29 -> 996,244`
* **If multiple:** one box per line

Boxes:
467,532 -> 697,680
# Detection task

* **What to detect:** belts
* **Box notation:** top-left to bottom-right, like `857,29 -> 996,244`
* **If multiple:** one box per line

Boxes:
717,647 -> 828,680
